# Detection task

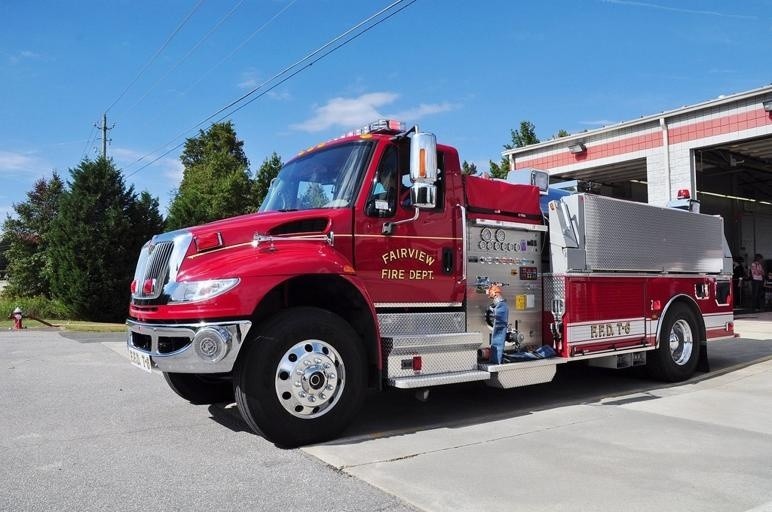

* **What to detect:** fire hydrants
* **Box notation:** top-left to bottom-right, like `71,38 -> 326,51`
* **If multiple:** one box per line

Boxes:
9,307 -> 25,329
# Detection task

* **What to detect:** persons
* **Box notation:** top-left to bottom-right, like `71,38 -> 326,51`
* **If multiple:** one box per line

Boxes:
749,251 -> 766,313
367,153 -> 415,218
729,255 -> 746,309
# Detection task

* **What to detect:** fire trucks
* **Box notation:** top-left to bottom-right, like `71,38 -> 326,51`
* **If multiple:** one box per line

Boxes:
126,119 -> 738,449
